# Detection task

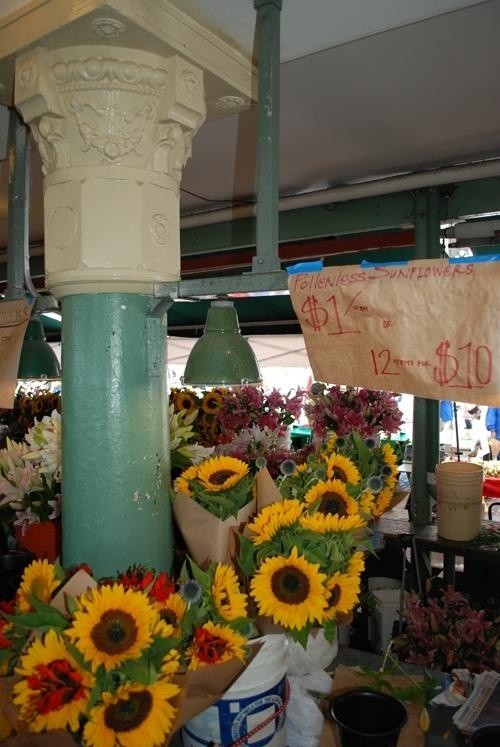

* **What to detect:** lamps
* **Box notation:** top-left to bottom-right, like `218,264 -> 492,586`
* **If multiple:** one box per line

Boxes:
181,293 -> 264,386
17,311 -> 60,381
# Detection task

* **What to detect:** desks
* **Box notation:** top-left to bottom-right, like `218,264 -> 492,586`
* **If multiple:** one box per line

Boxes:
360,517 -> 499,643
340,646 -> 463,747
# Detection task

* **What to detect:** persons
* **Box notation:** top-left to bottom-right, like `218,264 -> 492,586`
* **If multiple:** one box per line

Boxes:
439,398 -> 500,460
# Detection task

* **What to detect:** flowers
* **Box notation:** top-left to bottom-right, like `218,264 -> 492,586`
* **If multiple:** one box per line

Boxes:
0,553 -> 247,747
178,378 -> 418,633
2,373 -> 63,531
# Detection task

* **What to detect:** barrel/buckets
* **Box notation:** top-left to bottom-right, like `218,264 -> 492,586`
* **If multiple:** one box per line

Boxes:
433,460 -> 486,544
